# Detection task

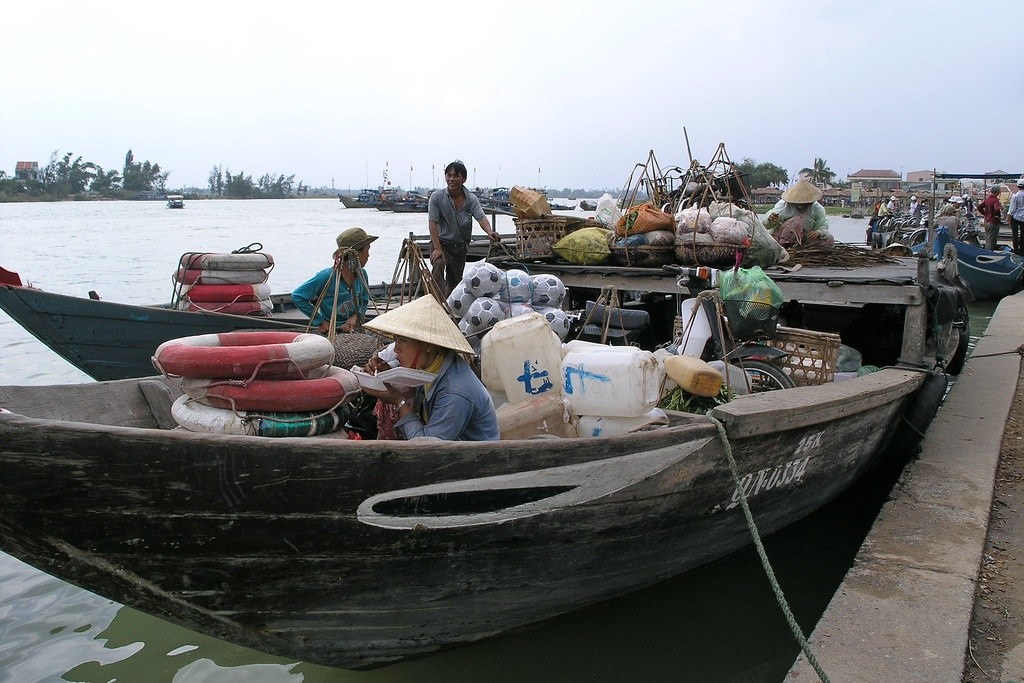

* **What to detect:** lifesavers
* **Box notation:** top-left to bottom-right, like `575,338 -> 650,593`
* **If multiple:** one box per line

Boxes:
171,394 -> 351,437
943,318 -> 972,377
177,285 -> 271,301
174,425 -> 350,439
154,331 -> 336,377
175,300 -> 274,315
173,269 -> 267,285
888,374 -> 948,461
180,365 -> 359,412
180,253 -> 274,269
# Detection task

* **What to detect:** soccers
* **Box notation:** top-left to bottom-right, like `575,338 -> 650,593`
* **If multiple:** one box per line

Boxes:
447,262 -> 571,375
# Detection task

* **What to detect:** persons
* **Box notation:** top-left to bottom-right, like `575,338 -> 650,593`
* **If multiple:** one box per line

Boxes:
935,192 -> 977,219
1005,178 -> 1024,256
909,196 -> 918,217
291,227 -> 380,333
762,177 -> 830,241
428,159 -> 501,299
360,293 -> 502,443
977,185 -> 1002,251
873,196 -> 896,217
921,198 -> 928,218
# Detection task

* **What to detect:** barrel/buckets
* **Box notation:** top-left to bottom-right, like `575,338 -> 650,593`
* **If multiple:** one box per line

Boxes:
481,311 -> 669,439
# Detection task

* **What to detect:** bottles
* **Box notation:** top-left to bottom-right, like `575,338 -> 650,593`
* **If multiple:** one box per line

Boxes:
662,355 -> 723,395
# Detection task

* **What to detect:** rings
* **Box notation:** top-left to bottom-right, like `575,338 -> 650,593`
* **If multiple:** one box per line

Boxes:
352,329 -> 354,332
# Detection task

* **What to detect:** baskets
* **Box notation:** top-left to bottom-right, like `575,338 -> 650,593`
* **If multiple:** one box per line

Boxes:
720,172 -> 751,197
674,240 -> 749,268
714,172 -> 741,195
514,216 -> 567,261
722,299 -> 778,339
608,234 -> 676,267
746,326 -> 841,392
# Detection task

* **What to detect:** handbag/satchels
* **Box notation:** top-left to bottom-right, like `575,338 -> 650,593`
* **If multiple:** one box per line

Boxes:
596,193 -> 622,229
719,265 -> 783,319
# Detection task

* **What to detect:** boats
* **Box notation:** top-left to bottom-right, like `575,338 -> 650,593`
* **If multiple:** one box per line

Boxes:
851,208 -> 869,219
0,265 -> 971,672
568,194 -> 576,200
580,200 -> 597,211
337,160 -> 577,214
842,214 -> 850,218
165,195 -> 185,209
909,225 -> 1024,298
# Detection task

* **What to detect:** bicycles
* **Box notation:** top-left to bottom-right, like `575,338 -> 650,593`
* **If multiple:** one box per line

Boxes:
878,210 -> 991,250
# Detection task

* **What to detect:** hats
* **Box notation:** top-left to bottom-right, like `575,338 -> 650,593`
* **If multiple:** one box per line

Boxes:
952,197 -> 963,204
781,179 -> 822,204
890,196 -> 895,201
911,196 -> 916,200
362,294 -> 475,354
333,228 -> 379,258
1016,179 -> 1024,187
948,196 -> 956,203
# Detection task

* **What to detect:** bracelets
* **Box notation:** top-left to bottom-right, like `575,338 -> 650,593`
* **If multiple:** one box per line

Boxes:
393,398 -> 407,413
